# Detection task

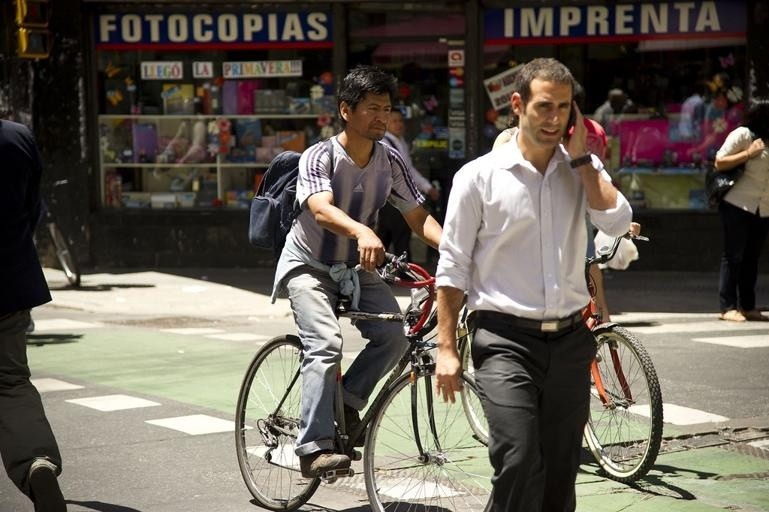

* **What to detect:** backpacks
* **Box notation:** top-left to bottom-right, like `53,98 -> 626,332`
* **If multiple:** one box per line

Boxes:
248,150 -> 302,251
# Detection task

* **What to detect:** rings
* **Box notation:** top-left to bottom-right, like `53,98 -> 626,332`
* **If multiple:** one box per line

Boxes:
439,382 -> 448,390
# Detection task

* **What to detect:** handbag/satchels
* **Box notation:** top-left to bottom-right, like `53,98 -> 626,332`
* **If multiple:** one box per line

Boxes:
701,128 -> 757,204
294,449 -> 352,477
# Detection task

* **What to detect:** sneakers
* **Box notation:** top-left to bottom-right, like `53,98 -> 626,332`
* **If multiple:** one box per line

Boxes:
27,454 -> 69,512
720,306 -> 768,322
335,398 -> 367,443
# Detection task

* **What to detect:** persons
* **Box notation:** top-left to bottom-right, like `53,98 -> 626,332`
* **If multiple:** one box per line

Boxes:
155,99 -> 212,191
715,101 -> 769,324
630,220 -> 643,238
0,120 -> 66,512
266,62 -> 443,479
589,78 -> 728,167
564,79 -> 615,324
429,57 -> 637,512
491,106 -> 523,152
381,104 -> 440,280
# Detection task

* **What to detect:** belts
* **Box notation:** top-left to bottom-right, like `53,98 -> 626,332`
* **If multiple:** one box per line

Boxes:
477,310 -> 586,333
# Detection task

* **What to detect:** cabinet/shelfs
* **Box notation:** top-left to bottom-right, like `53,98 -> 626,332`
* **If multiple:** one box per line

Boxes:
95,109 -> 340,218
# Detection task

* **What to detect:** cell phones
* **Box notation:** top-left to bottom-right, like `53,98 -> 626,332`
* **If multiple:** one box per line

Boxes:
569,108 -> 576,128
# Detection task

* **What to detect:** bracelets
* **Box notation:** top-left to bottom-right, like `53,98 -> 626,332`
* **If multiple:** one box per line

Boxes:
569,153 -> 592,170
746,148 -> 752,161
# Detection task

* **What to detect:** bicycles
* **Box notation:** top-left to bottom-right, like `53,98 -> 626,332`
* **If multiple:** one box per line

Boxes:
33,179 -> 82,289
